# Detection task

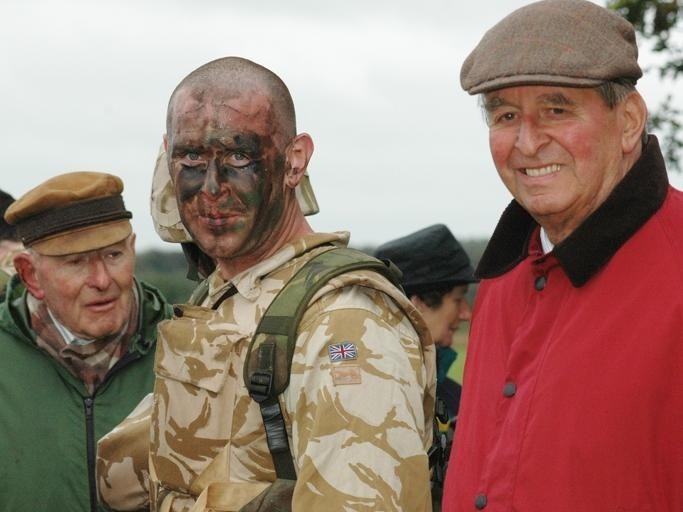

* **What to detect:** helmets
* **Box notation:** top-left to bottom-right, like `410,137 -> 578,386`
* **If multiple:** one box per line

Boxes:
151,141 -> 319,244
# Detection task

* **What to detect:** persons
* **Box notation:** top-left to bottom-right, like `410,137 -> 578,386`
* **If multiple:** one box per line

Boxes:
90,55 -> 436,511
0,171 -> 177,511
0,191 -> 30,305
437,2 -> 683,512
370,223 -> 481,510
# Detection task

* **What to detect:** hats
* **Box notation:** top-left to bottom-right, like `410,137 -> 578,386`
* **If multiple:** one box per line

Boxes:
4,170 -> 132,258
459,0 -> 645,96
0,191 -> 22,243
373,224 -> 479,290
